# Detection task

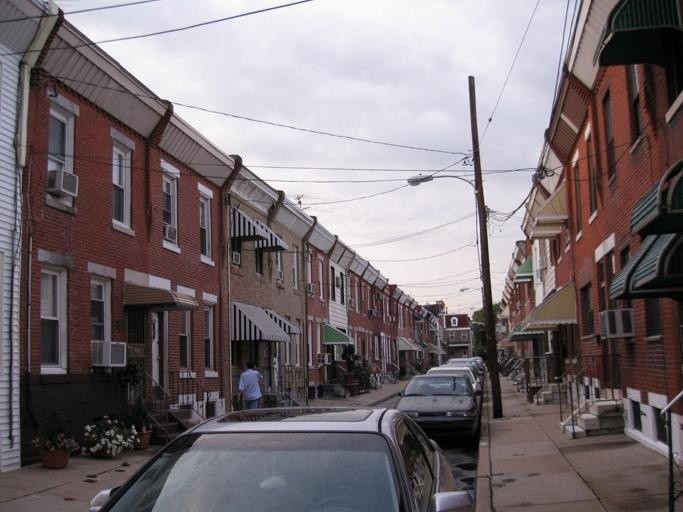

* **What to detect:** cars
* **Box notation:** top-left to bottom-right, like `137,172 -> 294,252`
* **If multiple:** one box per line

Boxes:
88,406 -> 474,512
397,356 -> 486,431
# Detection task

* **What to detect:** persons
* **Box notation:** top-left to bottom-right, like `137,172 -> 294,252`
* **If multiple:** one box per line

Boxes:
236,360 -> 264,409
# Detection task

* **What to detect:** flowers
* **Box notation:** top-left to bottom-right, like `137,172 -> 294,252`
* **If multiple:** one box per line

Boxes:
31,412 -> 141,458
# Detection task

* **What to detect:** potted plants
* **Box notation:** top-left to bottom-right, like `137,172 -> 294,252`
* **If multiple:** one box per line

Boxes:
341,344 -> 355,384
124,394 -> 155,448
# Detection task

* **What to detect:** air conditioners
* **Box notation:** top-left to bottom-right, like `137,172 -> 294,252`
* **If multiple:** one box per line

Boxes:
46,168 -> 79,198
307,283 -> 314,293
277,271 -> 283,280
91,341 -> 127,367
232,252 -> 240,264
348,299 -> 355,307
163,222 -> 176,243
599,308 -> 636,339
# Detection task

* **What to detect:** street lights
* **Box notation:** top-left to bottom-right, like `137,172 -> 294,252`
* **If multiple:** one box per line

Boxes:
408,170 -> 504,422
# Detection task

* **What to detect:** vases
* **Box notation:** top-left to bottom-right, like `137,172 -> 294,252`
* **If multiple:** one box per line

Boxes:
39,448 -> 71,468
